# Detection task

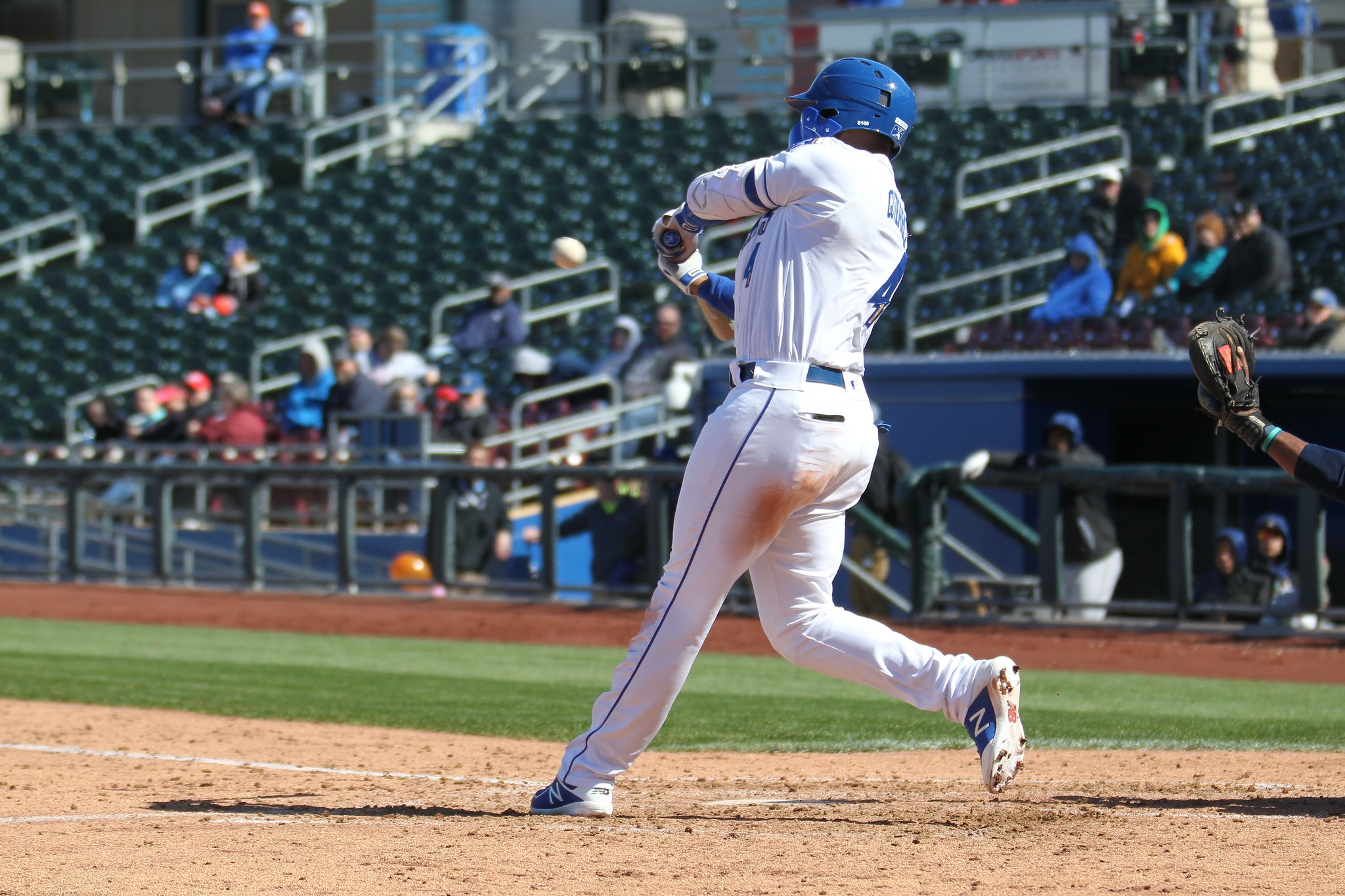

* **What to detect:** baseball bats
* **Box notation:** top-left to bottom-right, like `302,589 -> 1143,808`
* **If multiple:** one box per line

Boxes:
660,228 -> 682,249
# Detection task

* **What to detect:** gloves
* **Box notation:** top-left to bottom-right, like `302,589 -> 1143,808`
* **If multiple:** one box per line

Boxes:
874,419 -> 891,434
650,200 -> 705,264
657,248 -> 708,296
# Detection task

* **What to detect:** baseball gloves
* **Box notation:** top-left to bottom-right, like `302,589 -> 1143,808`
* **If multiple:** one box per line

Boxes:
1187,319 -> 1262,432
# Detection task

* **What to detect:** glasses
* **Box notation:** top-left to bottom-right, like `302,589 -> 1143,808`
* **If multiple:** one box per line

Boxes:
1251,524 -> 1280,540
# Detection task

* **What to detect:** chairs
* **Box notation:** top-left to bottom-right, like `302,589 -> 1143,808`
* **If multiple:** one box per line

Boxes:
0,88 -> 1344,501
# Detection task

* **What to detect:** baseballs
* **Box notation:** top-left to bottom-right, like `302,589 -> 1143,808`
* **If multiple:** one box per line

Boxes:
547,236 -> 589,270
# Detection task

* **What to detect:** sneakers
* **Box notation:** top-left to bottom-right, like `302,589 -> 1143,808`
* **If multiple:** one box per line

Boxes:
530,773 -> 614,817
964,656 -> 1027,795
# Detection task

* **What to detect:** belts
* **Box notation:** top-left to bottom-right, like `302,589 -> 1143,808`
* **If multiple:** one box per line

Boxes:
728,360 -> 845,387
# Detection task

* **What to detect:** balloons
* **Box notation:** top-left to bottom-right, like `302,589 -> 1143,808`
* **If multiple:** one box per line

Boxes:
391,552 -> 431,593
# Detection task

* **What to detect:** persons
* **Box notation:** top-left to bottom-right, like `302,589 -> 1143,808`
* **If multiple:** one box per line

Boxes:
1221,0 -> 1283,152
522,476 -> 648,604
961,414 -> 1123,621
1113,199 -> 1188,317
1195,513 -> 1330,629
1030,234 -> 1113,325
83,371 -> 267,531
580,302 -> 698,462
424,443 -> 530,599
153,236 -> 269,319
1167,211 -> 1231,295
203,0 -> 316,130
1188,317 -> 1345,505
531,56 -> 1024,814
268,269 -> 525,528
850,402 -> 911,622
1279,287 -> 1345,353
1185,202 -> 1293,323
1079,165 -> 1142,272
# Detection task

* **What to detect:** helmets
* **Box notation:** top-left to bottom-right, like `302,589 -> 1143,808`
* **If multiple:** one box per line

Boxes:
1044,412 -> 1083,447
782,56 -> 917,162
